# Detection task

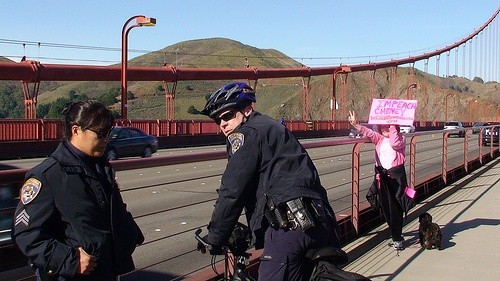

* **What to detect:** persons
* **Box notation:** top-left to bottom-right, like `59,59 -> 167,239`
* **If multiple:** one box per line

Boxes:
11,100 -> 144,281
197,82 -> 341,281
348,111 -> 408,250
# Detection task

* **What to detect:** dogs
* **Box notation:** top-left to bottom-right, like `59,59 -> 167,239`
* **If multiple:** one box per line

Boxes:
416,211 -> 442,250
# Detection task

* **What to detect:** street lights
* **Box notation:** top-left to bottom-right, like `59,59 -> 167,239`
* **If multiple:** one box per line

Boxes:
408,83 -> 417,100
121,15 -> 157,126
445,93 -> 455,122
469,99 -> 477,126
331,66 -> 351,122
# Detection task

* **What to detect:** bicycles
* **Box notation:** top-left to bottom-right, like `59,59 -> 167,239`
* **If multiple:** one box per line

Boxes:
193,221 -> 372,281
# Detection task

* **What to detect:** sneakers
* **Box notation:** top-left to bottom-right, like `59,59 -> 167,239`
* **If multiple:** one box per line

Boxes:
389,236 -> 405,246
394,240 -> 404,249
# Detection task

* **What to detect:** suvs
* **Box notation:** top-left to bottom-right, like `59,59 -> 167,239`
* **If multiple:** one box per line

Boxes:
443,121 -> 466,137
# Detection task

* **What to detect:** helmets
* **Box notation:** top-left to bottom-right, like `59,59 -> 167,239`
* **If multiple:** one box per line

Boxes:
204,82 -> 256,118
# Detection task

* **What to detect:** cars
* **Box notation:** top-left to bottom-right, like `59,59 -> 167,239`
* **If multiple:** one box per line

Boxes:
479,125 -> 500,146
349,123 -> 379,143
472,123 -> 484,134
400,125 -> 415,134
105,125 -> 160,162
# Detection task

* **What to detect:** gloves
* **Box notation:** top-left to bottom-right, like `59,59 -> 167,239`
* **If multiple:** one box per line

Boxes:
196,236 -> 225,254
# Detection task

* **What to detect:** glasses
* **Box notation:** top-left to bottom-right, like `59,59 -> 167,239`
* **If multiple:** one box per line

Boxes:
215,107 -> 241,125
71,123 -> 112,138
